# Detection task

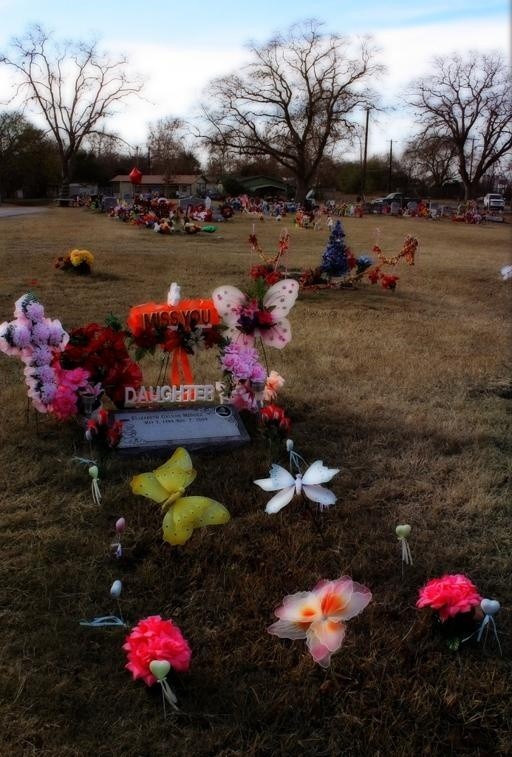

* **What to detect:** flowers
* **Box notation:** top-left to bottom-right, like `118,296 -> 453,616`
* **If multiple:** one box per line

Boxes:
55,249 -> 95,271
120,614 -> 192,688
268,573 -> 376,662
420,575 -> 482,623
319,221 -> 374,277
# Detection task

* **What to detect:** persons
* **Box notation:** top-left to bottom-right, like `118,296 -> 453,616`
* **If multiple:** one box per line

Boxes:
326,214 -> 333,232
261,199 -> 270,215
274,200 -> 281,217
327,203 -> 348,216
353,204 -> 361,217
311,204 -> 323,231
465,207 -> 474,224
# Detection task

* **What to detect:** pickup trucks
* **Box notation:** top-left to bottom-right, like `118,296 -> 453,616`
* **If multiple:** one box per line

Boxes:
373,191 -> 421,205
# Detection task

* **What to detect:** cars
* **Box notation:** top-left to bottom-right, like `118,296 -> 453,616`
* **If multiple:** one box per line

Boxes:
483,193 -> 507,210
